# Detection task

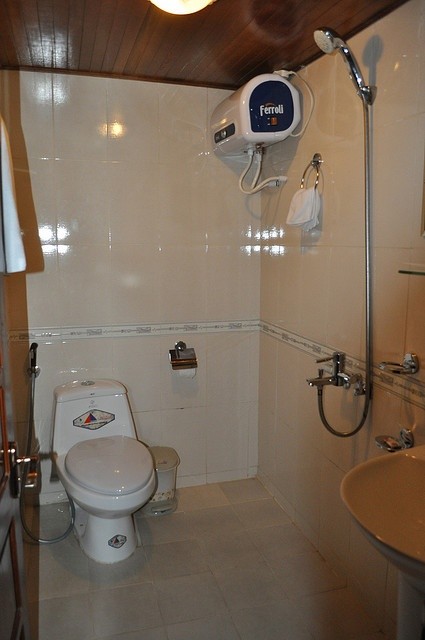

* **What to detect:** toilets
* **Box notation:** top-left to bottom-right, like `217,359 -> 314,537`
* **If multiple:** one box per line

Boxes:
49,378 -> 157,566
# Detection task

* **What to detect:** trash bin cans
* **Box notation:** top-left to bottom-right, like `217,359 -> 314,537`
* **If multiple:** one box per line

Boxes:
140,446 -> 180,517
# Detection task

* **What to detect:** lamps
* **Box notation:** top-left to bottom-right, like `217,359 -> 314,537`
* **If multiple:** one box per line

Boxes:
149,0 -> 216,16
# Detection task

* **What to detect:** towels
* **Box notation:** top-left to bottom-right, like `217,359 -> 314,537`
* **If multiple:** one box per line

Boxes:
0,117 -> 27,274
286,187 -> 320,233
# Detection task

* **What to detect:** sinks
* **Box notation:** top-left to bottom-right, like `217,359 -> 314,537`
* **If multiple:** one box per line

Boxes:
340,443 -> 425,580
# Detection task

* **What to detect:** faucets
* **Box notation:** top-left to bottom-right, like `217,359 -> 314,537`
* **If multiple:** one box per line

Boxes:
306,352 -> 346,386
